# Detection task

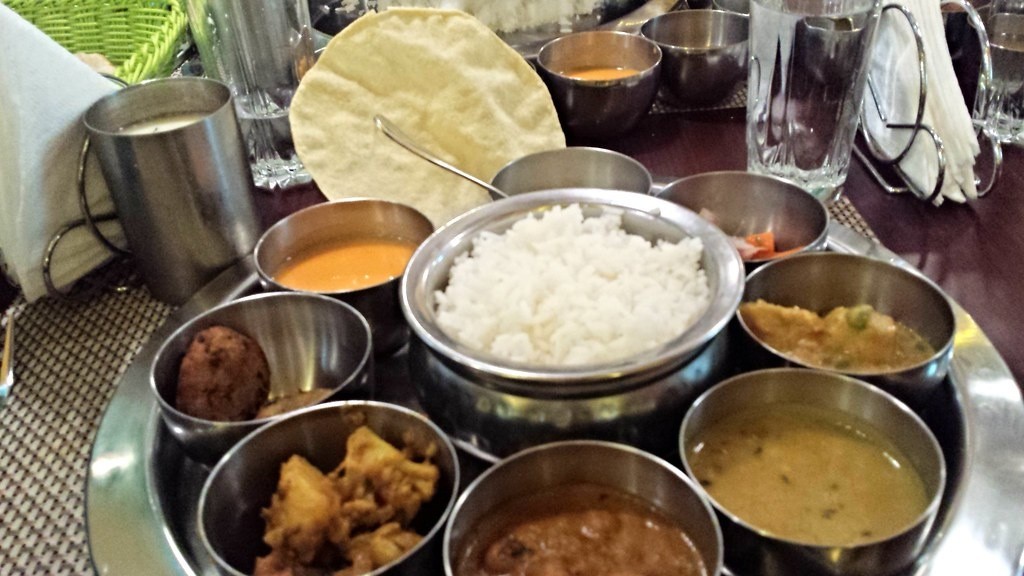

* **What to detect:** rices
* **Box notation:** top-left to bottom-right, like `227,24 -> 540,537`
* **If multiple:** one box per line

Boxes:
434,201 -> 710,367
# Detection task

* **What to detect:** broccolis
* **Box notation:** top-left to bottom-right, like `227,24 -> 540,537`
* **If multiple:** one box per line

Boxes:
251,423 -> 442,576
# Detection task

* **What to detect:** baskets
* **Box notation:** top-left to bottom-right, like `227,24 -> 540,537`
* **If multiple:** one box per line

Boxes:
1,0 -> 200,96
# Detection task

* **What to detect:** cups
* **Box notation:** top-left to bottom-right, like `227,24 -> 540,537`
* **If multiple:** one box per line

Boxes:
971,0 -> 1024,147
746,0 -> 883,206
185,0 -> 317,190
74,76 -> 263,305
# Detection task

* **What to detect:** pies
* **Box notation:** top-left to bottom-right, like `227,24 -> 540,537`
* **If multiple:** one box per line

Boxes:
289,8 -> 568,229
179,322 -> 338,424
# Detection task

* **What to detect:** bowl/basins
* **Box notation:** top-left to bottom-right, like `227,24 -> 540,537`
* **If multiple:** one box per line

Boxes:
941,2 -> 969,56
442,439 -> 723,576
678,368 -> 948,576
641,9 -> 751,107
736,251 -> 957,414
968,6 -> 1024,32
537,30 -> 663,144
656,170 -> 829,272
486,146 -> 653,198
254,196 -> 436,363
197,399 -> 461,576
397,187 -> 747,466
314,0 -> 681,61
149,291 -> 377,473
